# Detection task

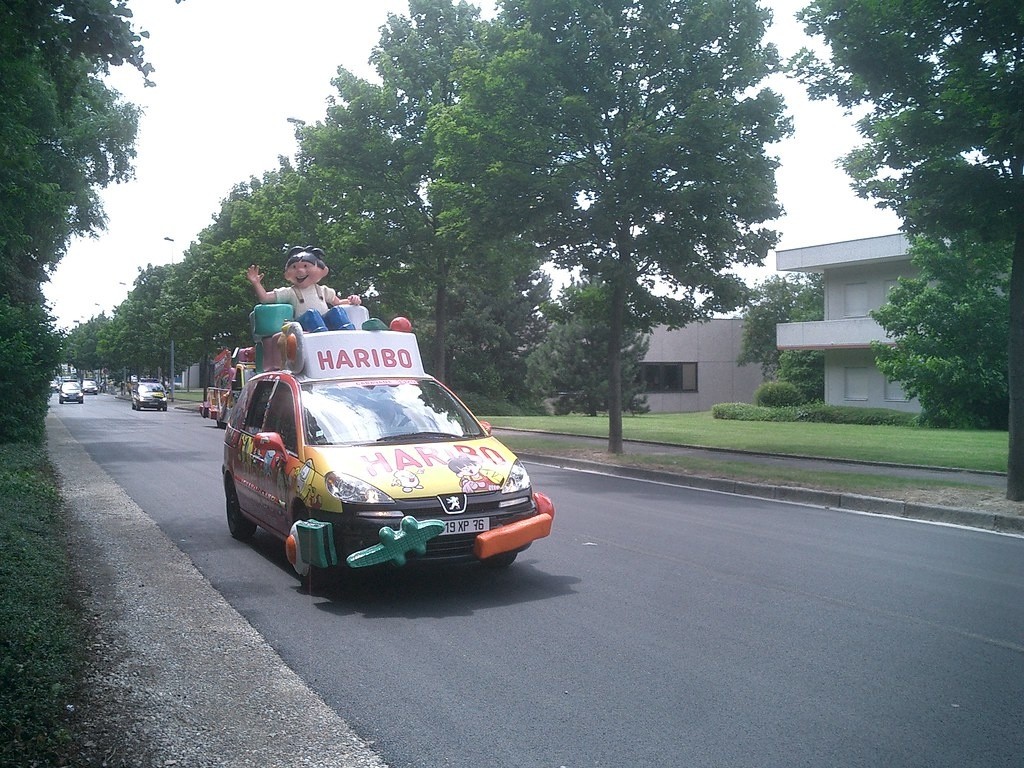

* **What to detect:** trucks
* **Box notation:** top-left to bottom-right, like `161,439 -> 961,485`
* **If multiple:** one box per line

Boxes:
198,361 -> 258,429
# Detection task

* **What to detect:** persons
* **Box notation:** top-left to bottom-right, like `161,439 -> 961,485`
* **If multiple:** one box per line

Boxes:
246,245 -> 362,333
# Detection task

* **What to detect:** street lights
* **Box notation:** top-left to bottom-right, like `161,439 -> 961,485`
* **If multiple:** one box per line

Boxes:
164,236 -> 177,403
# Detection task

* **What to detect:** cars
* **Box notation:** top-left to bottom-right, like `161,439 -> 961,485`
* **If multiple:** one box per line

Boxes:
81,378 -> 99,396
50,381 -> 59,394
132,377 -> 170,412
221,305 -> 554,583
59,379 -> 84,404
